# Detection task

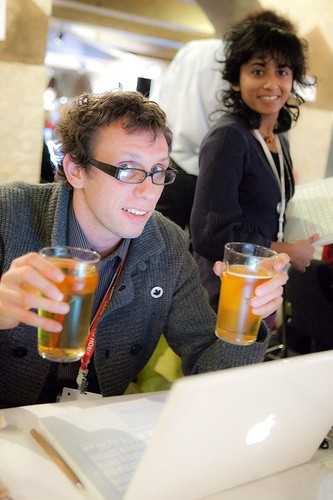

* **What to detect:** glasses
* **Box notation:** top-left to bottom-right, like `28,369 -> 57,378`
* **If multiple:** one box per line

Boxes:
77,148 -> 179,186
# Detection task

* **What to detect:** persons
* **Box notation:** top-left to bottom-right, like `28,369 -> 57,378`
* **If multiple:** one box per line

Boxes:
189,11 -> 333,355
0,90 -> 288,410
152,39 -> 234,232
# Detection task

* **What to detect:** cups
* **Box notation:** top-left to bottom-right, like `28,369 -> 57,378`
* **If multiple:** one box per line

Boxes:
38,246 -> 102,362
215,243 -> 278,346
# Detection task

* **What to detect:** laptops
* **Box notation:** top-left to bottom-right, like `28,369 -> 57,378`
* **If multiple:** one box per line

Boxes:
36,349 -> 333,500
283,176 -> 333,248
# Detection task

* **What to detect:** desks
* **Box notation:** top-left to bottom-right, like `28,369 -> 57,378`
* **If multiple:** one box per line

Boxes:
0,389 -> 333,500
